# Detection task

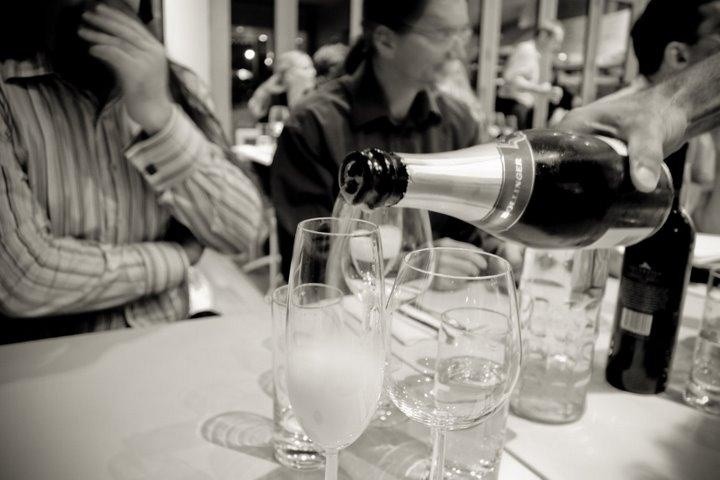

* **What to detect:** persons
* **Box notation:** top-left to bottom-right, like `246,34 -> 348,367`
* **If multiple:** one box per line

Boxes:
574,1 -> 720,240
494,16 -> 567,134
272,0 -> 508,303
554,48 -> 720,192
1,2 -> 271,345
248,41 -> 493,138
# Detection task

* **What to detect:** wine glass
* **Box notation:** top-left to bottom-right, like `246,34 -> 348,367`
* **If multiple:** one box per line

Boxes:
287,215 -> 389,478
379,245 -> 519,479
329,193 -> 437,429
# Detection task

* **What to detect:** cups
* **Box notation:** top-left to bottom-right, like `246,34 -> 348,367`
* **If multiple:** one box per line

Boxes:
272,283 -> 343,472
682,267 -> 720,416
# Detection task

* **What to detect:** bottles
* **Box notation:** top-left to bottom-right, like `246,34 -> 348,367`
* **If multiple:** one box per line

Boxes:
511,246 -> 611,424
604,142 -> 696,394
338,127 -> 675,250
